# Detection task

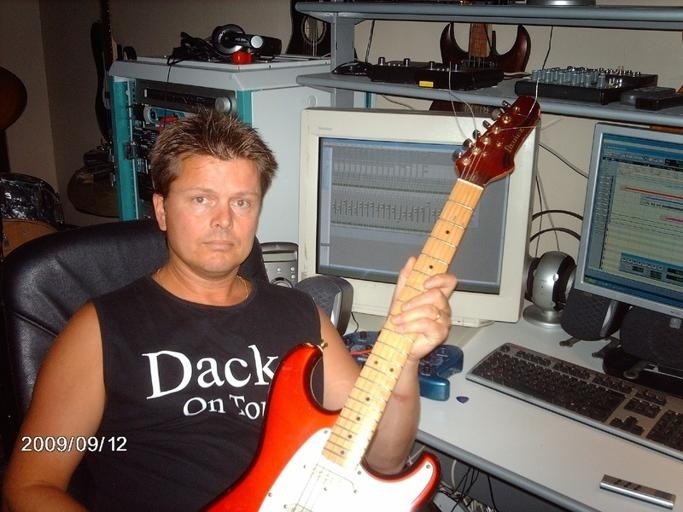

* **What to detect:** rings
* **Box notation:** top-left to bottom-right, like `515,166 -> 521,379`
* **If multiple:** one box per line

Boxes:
435,309 -> 441,322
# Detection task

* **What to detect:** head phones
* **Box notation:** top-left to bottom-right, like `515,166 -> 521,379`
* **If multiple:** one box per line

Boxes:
210,23 -> 283,62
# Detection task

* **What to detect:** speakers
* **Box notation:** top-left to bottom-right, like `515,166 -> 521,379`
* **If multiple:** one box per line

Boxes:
560,280 -> 629,340
296,276 -> 354,336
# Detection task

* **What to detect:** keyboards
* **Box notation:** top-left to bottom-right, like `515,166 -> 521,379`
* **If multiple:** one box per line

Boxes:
466,340 -> 683,462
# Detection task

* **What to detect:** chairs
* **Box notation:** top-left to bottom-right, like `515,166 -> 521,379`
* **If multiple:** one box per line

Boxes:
0,219 -> 269,465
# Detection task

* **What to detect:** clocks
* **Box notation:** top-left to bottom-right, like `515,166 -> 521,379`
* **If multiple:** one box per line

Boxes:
284,0 -> 357,60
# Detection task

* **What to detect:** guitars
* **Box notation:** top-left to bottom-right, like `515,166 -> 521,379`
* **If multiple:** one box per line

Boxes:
210,95 -> 540,511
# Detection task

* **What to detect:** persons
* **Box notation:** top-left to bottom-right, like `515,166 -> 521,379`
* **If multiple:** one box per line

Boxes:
1,109 -> 458,512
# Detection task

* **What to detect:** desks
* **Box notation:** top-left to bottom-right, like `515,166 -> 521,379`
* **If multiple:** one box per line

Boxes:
295,0 -> 683,512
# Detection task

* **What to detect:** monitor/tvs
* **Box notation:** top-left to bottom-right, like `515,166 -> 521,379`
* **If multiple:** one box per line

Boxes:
574,122 -> 682,365
299,105 -> 541,327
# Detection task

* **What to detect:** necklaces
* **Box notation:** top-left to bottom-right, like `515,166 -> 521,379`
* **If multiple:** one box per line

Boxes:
156,266 -> 249,300
